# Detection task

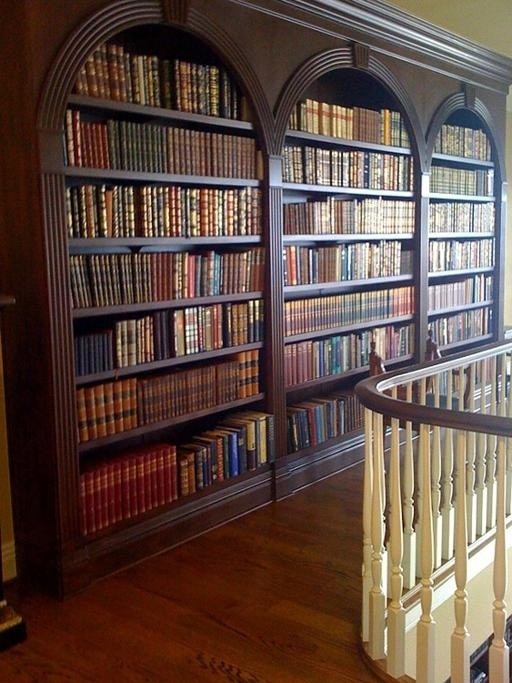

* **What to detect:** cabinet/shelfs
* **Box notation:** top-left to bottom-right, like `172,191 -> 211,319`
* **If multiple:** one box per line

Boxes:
426,90 -> 504,413
61,11 -> 277,588
276,42 -> 427,494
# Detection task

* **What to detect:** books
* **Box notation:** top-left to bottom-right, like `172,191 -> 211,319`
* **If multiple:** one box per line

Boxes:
427,274 -> 494,311
79,409 -> 274,537
283,193 -> 416,235
430,165 -> 494,197
287,392 -> 364,454
283,238 -> 402,286
428,201 -> 495,234
74,300 -> 266,378
63,184 -> 264,240
284,323 -> 416,388
427,306 -> 494,347
280,145 -> 415,192
428,237 -> 495,272
286,97 -> 411,148
431,124 -> 491,161
70,245 -> 265,309
283,285 -> 415,338
74,42 -> 249,122
63,108 -> 256,179
76,350 -> 268,444
